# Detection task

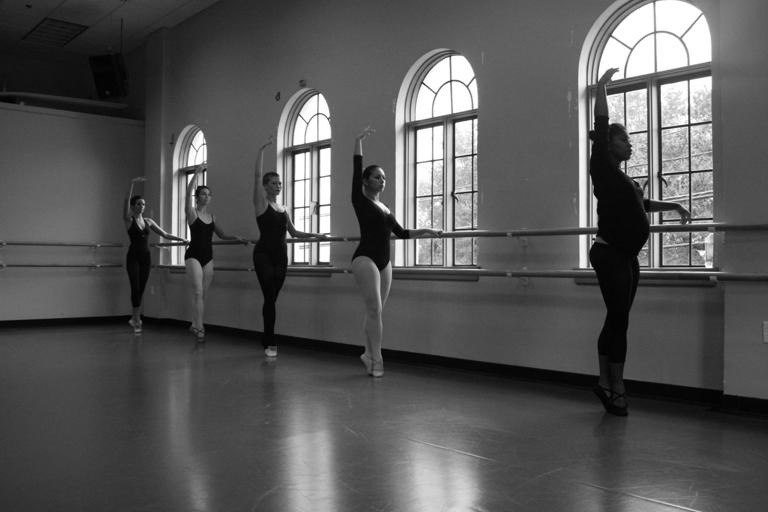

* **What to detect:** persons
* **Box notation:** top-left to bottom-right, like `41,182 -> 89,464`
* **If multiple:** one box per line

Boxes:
349,125 -> 443,377
123,176 -> 189,333
253,135 -> 330,357
589,67 -> 693,417
183,160 -> 249,343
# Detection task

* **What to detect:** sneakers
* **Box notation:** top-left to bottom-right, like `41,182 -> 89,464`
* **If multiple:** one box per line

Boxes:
192,327 -> 206,338
595,384 -> 629,417
264,347 -> 278,357
359,353 -> 386,378
127,319 -> 143,333
